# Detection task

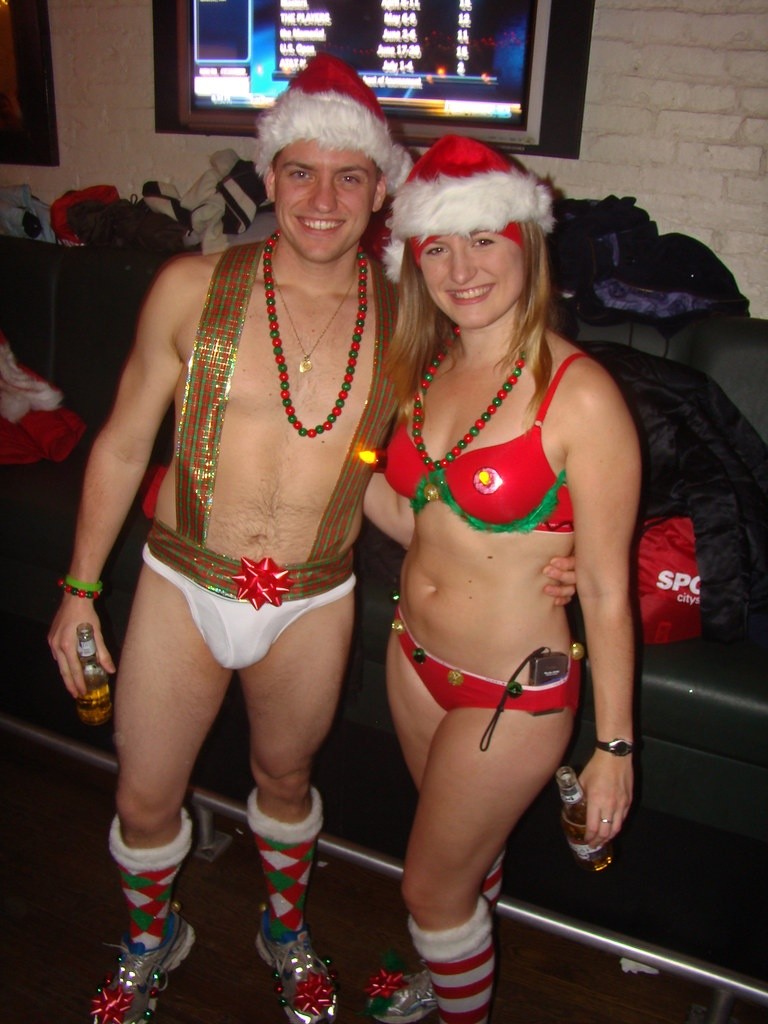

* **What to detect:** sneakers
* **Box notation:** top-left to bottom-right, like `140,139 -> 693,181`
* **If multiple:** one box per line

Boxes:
364,960 -> 439,1024
256,904 -> 338,1024
90,911 -> 196,1024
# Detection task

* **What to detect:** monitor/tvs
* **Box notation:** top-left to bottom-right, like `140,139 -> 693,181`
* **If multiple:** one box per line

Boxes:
175,0 -> 551,152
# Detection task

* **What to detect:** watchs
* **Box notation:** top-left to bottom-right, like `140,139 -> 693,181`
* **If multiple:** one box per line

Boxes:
594,737 -> 636,758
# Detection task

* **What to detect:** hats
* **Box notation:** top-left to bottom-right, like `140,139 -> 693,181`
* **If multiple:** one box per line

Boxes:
257,52 -> 413,194
383,134 -> 556,284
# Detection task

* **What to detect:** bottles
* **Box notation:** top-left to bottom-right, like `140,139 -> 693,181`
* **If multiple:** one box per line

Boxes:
68,624 -> 111,726
557,766 -> 613,870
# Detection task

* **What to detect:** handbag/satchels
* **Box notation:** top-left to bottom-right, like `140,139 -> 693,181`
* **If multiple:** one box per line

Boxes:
630,513 -> 703,644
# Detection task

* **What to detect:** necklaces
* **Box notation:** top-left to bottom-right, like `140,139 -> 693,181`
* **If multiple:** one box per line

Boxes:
272,255 -> 358,373
412,324 -> 531,469
261,228 -> 368,439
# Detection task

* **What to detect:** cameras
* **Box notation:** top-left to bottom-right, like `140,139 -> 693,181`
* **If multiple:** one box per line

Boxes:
530,652 -> 568,716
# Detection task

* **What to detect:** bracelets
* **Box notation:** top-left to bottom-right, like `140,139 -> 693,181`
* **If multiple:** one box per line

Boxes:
55,576 -> 106,601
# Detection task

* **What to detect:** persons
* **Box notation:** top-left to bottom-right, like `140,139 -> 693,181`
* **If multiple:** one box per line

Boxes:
47,40 -> 636,1024
